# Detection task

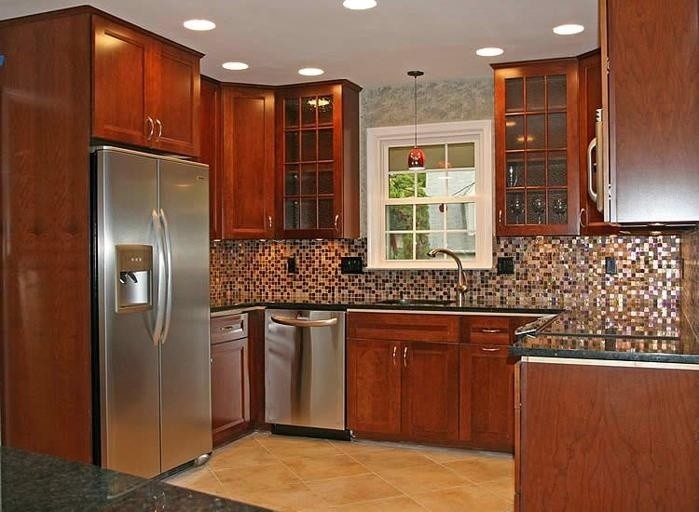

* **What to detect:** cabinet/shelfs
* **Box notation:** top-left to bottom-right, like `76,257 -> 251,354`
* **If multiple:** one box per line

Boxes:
220,86 -> 277,240
512,364 -> 698,510
489,2 -> 698,239
90,16 -> 203,157
345,307 -> 558,446
195,78 -> 219,240
276,82 -> 343,239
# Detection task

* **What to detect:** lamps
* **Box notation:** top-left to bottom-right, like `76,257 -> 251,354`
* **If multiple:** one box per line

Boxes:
408,71 -> 426,170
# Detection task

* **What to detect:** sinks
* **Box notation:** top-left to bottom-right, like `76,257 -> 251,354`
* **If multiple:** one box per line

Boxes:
373,299 -> 455,306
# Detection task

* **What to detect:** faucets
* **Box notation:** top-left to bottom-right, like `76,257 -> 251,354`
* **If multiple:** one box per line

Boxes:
427,248 -> 469,307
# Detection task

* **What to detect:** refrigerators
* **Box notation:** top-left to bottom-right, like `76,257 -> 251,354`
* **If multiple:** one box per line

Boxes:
88,144 -> 215,481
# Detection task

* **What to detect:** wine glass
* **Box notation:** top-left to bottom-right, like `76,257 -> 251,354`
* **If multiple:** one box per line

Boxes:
532,198 -> 545,224
509,200 -> 524,223
553,198 -> 568,224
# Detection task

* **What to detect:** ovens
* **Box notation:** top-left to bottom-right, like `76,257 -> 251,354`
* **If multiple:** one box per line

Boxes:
262,308 -> 347,433
